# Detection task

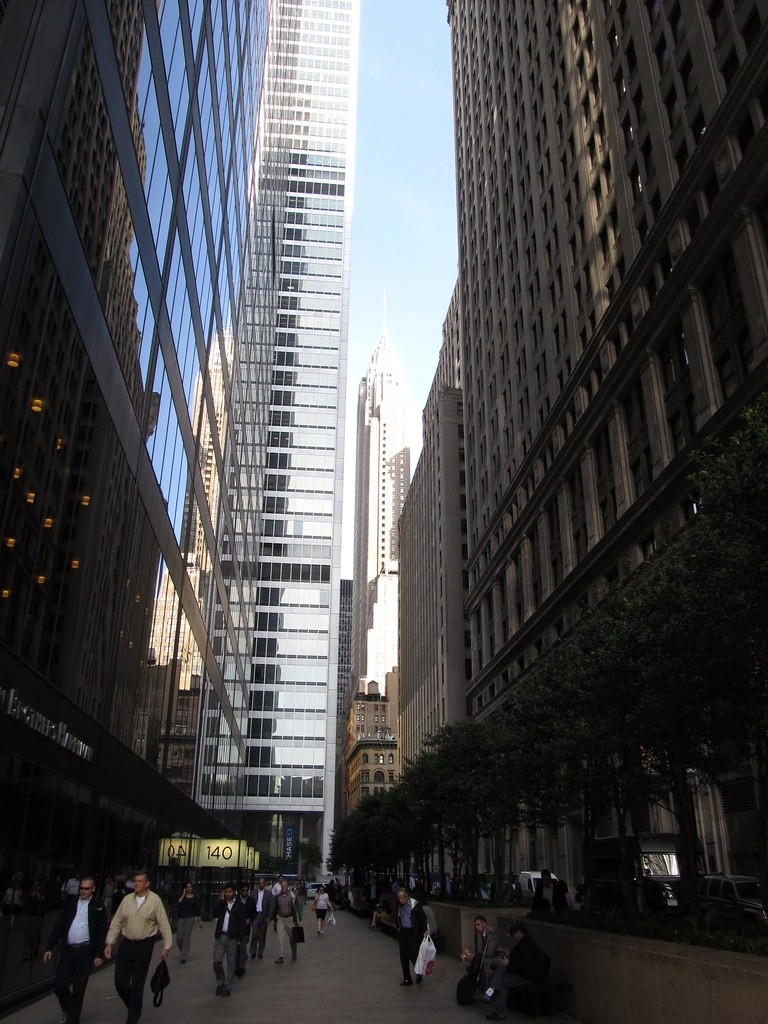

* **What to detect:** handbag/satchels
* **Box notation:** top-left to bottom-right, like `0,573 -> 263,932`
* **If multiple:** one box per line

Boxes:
311,893 -> 319,911
328,912 -> 336,926
150,957 -> 170,1007
292,922 -> 305,944
468,952 -> 483,980
414,934 -> 436,975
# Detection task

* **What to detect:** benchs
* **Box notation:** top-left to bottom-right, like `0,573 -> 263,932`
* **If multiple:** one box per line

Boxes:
348,904 -> 373,918
466,966 -> 574,1019
376,917 -> 445,954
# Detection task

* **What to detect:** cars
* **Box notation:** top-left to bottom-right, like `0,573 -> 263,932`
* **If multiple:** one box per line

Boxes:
306,883 -> 329,898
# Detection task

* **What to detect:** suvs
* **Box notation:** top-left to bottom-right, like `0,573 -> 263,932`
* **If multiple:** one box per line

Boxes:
697,874 -> 767,922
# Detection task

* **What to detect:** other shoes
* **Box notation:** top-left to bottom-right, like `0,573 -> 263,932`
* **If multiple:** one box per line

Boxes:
416,973 -> 423,984
485,1012 -> 505,1021
59,1012 -> 69,1024
258,955 -> 263,960
399,978 -> 413,986
215,983 -> 231,997
234,967 -> 245,978
250,953 -> 256,959
317,931 -> 324,935
370,922 -> 376,927
274,957 -> 284,964
292,951 -> 297,961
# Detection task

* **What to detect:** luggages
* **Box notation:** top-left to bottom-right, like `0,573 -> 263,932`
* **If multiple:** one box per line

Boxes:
456,933 -> 491,1005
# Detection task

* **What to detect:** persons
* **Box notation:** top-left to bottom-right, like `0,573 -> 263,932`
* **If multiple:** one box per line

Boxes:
212,884 -> 246,997
512,876 -> 523,898
234,883 -> 258,976
329,875 -> 416,927
463,915 -> 543,1019
398,888 -> 437,986
250,874 -> 307,964
532,869 -> 569,912
103,870 -> 172,1024
3,881 -> 45,964
176,883 -> 200,964
315,886 -> 333,934
446,877 -> 464,896
42,876 -> 106,1024
65,872 -> 80,900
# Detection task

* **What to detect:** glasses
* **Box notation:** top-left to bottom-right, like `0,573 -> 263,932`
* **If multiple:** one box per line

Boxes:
79,887 -> 94,890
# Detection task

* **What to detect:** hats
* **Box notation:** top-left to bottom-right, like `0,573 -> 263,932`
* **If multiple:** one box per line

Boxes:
506,918 -> 524,937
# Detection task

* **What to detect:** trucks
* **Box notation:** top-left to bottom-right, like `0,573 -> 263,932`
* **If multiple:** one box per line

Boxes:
518,871 -> 559,898
